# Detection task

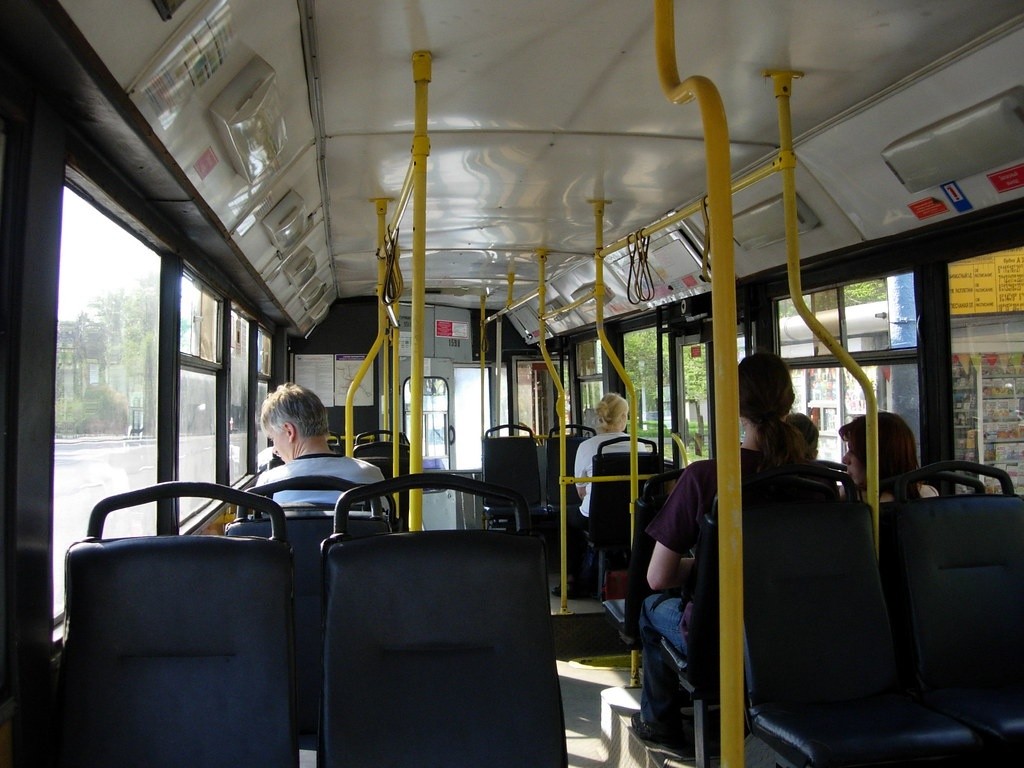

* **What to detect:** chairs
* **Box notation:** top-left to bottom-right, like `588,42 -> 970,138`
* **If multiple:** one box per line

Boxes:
579,437 -> 664,601
894,461 -> 1024,768
546,424 -> 596,534
323,473 -> 569,768
267,430 -> 344,468
57,480 -> 302,768
481,424 -> 557,531
878,470 -> 987,696
354,430 -> 410,529
750,462 -> 988,768
221,475 -> 400,752
660,476 -> 838,768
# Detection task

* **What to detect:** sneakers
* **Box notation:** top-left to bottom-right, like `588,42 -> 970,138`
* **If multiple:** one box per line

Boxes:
630,711 -> 689,750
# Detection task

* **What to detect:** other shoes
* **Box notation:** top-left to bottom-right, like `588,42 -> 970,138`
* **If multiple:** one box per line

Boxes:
551,583 -> 574,598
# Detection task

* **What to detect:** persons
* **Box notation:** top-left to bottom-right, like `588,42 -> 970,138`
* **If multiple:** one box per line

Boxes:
254,381 -> 390,512
551,392 -> 648,599
632,353 -> 944,749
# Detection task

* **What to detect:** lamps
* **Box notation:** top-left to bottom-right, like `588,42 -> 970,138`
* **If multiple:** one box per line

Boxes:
311,300 -> 330,325
281,245 -> 318,289
301,277 -> 326,307
537,300 -> 569,322
733,192 -> 820,253
206,56 -> 289,181
262,188 -> 309,251
571,284 -> 610,308
877,86 -> 1024,193
